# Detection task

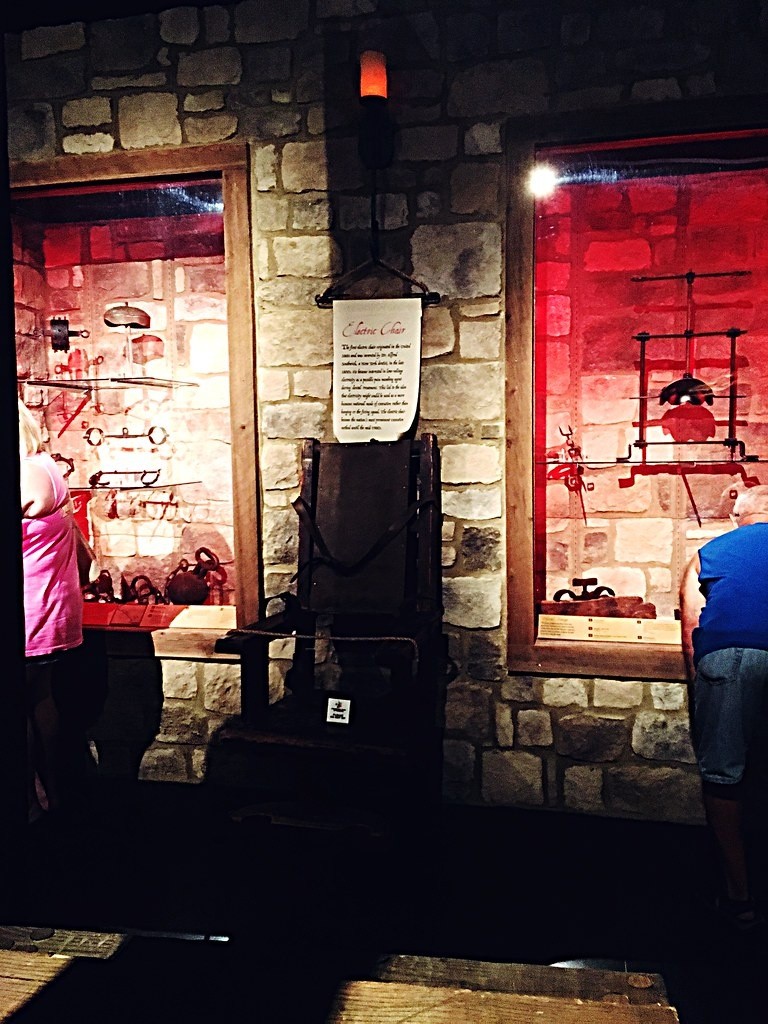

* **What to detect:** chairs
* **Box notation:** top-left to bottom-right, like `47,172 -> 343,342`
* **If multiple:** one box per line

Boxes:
204,435 -> 446,878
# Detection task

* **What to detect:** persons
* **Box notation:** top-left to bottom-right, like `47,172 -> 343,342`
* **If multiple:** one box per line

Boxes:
679,483 -> 767,932
19,399 -> 85,832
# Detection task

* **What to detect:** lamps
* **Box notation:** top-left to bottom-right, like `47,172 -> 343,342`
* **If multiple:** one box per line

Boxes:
355,51 -> 395,133
